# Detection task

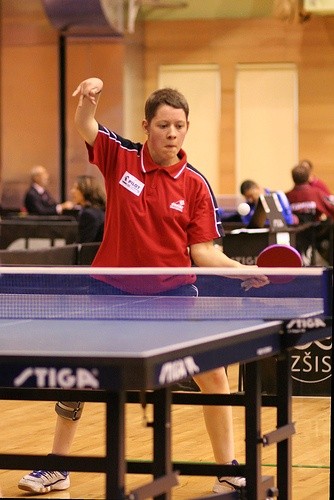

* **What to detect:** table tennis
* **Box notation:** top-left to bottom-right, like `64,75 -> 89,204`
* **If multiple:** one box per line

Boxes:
237,203 -> 250,216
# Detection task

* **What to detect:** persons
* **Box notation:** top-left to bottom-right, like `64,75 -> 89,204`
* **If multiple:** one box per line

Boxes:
23,158 -> 334,246
17,77 -> 269,494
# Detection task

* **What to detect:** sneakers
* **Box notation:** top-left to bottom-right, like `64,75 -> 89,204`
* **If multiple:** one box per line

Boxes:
211,460 -> 248,496
18,454 -> 71,494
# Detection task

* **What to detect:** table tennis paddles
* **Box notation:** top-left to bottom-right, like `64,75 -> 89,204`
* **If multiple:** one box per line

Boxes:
241,245 -> 302,288
